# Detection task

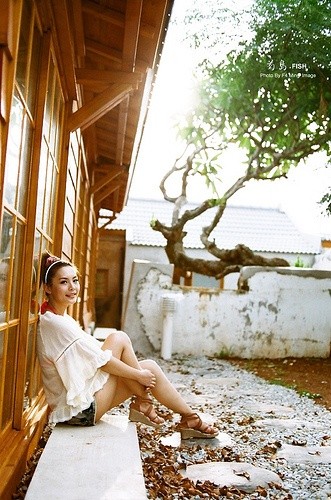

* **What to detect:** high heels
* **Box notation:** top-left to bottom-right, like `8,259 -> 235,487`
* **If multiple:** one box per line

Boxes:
178,413 -> 220,440
129,395 -> 165,429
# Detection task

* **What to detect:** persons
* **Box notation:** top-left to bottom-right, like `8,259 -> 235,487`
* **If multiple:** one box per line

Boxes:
36,252 -> 219,440
0,257 -> 39,324
